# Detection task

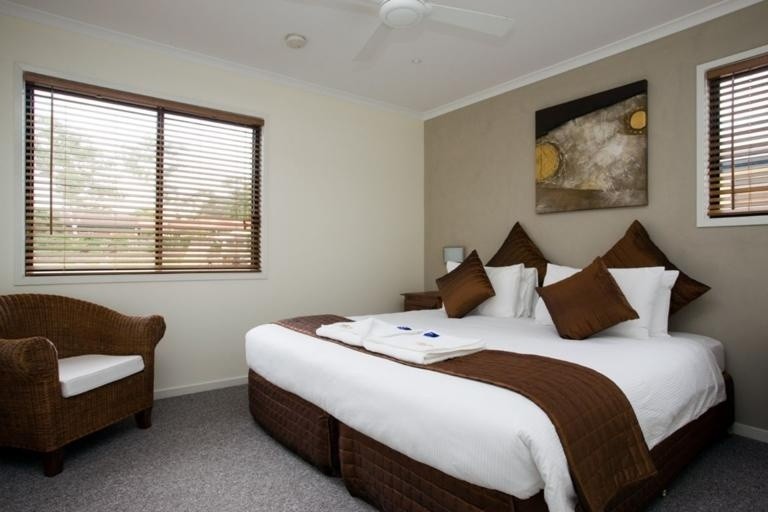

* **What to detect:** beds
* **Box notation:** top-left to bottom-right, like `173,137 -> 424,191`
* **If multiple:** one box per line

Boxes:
245,261 -> 738,511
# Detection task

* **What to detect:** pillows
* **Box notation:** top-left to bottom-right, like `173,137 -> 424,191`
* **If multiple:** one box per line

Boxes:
534,262 -> 666,337
433,248 -> 496,319
534,255 -> 639,339
600,219 -> 713,317
486,220 -> 551,287
519,267 -> 538,319
443,261 -> 527,320
647,269 -> 680,337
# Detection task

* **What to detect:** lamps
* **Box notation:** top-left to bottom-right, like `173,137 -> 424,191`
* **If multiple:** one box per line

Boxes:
441,245 -> 465,274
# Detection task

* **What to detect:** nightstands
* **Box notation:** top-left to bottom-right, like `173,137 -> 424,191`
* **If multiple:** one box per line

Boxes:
400,288 -> 443,314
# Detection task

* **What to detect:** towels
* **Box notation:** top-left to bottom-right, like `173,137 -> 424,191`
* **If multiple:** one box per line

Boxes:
314,310 -> 426,347
361,327 -> 490,365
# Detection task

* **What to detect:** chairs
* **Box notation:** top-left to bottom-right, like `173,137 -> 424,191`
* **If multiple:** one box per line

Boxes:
0,293 -> 166,477
